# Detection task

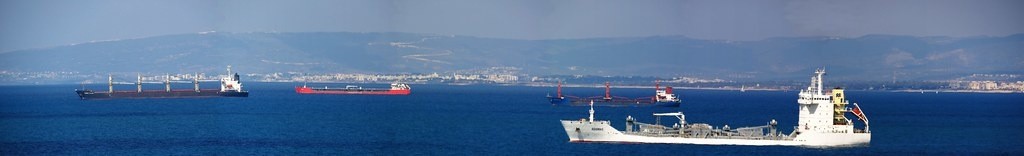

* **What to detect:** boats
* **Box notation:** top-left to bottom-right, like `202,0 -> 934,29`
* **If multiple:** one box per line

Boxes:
560,66 -> 873,150
545,78 -> 682,107
72,65 -> 249,98
295,80 -> 411,94
